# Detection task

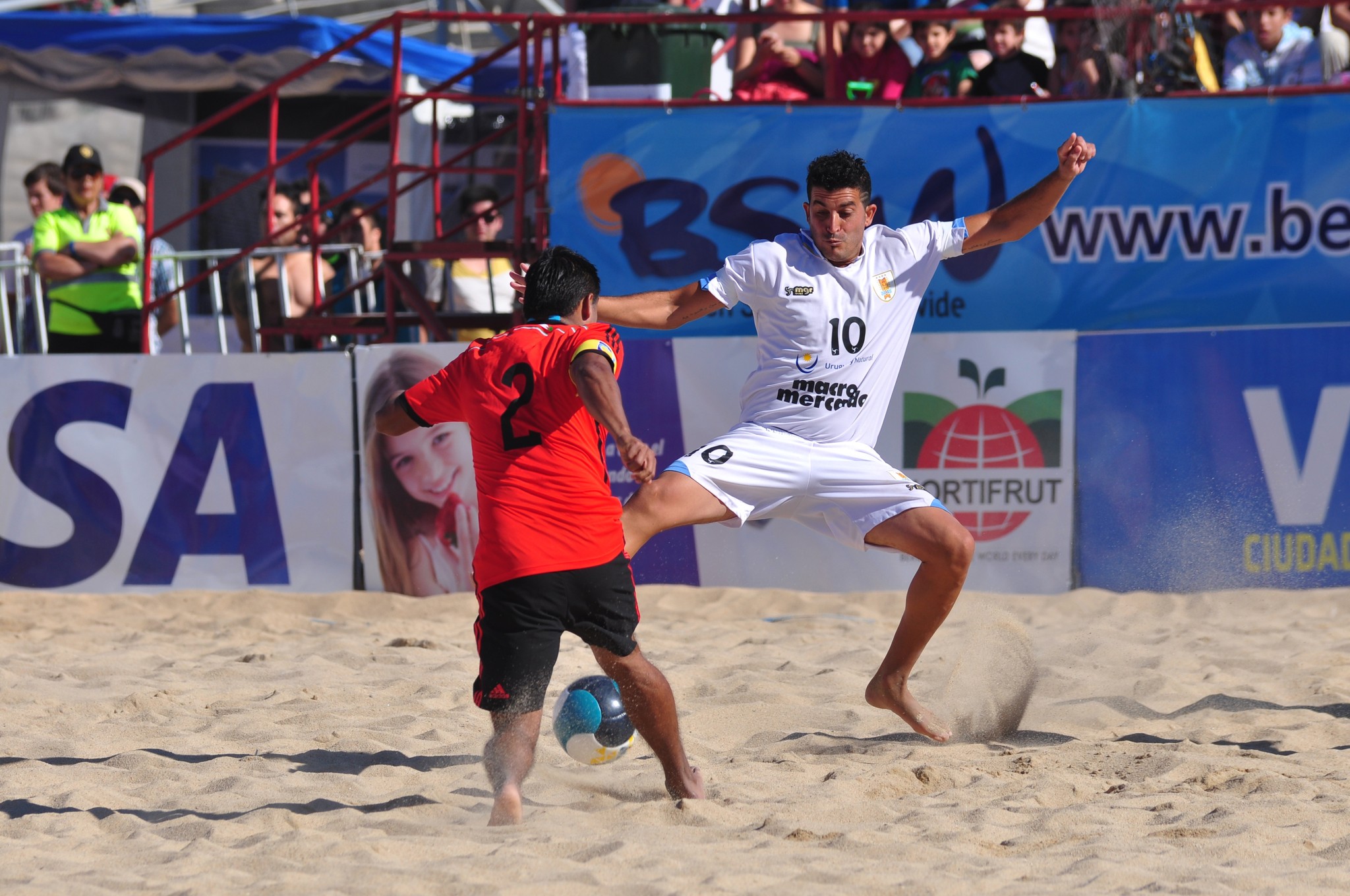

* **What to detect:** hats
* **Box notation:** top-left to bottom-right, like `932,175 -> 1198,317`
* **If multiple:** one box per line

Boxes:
64,144 -> 103,171
109,177 -> 146,204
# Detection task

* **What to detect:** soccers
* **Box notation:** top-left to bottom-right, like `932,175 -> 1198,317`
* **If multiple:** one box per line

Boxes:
551,675 -> 637,765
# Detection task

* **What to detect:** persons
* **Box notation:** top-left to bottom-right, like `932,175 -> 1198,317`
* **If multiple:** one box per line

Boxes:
727,0 -> 1350,100
106,177 -> 181,354
288,178 -> 346,268
31,142 -> 146,355
226,182 -> 342,355
508,131 -> 1097,745
2,160 -> 71,356
373,247 -> 705,827
333,202 -> 418,348
411,185 -> 533,343
363,349 -> 479,598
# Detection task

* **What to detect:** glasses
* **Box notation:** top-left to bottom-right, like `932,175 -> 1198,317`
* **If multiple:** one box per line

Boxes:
464,212 -> 500,224
110,193 -> 141,207
71,168 -> 97,179
